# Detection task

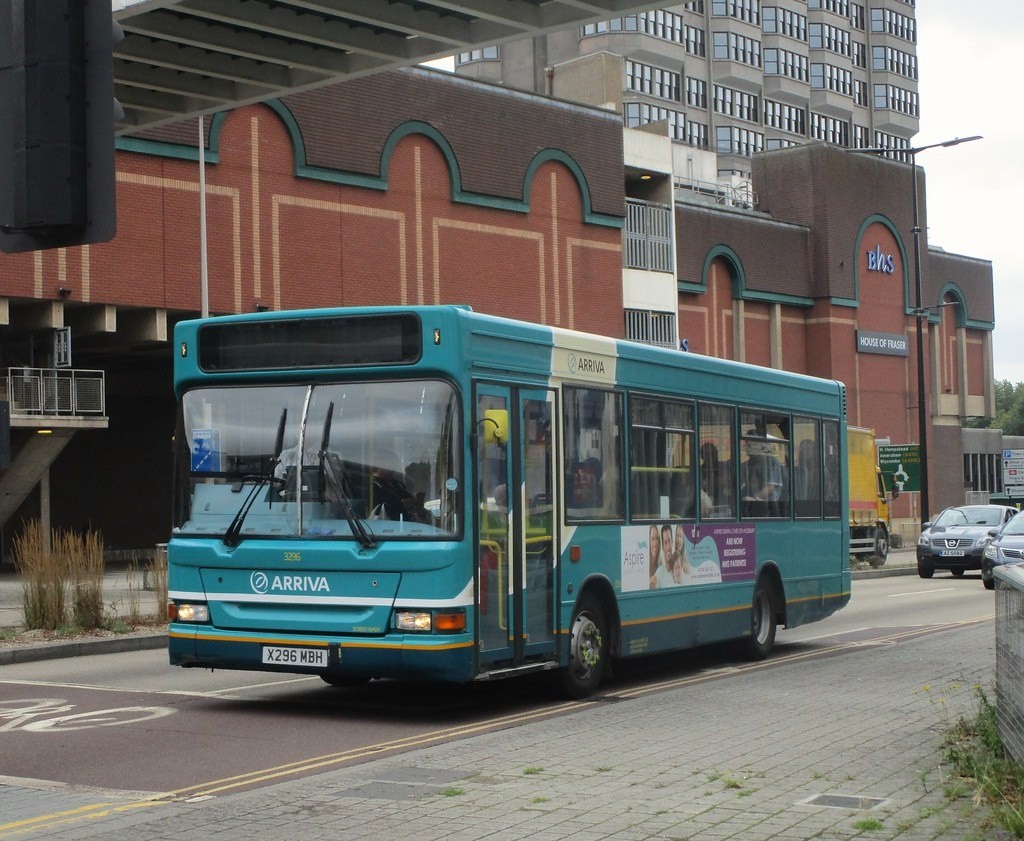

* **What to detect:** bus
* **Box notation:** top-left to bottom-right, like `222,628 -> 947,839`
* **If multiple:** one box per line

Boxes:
167,301 -> 853,706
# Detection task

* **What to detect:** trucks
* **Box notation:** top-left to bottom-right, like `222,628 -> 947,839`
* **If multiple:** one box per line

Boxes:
671,419 -> 891,568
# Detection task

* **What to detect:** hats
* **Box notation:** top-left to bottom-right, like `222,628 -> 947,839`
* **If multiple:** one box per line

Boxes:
578,457 -> 602,482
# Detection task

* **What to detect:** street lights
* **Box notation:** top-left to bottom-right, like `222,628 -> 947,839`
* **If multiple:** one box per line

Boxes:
846,135 -> 987,520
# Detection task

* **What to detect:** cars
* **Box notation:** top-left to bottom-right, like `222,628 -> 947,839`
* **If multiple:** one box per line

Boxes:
916,503 -> 1019,579
980,509 -> 1024,590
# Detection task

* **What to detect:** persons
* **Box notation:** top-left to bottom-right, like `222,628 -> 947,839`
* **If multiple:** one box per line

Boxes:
740,429 -> 783,502
365,449 -> 416,523
274,408 -> 343,501
648,525 -> 695,590
797,439 -> 832,502
570,457 -> 602,509
699,442 -> 735,506
670,465 -> 712,518
